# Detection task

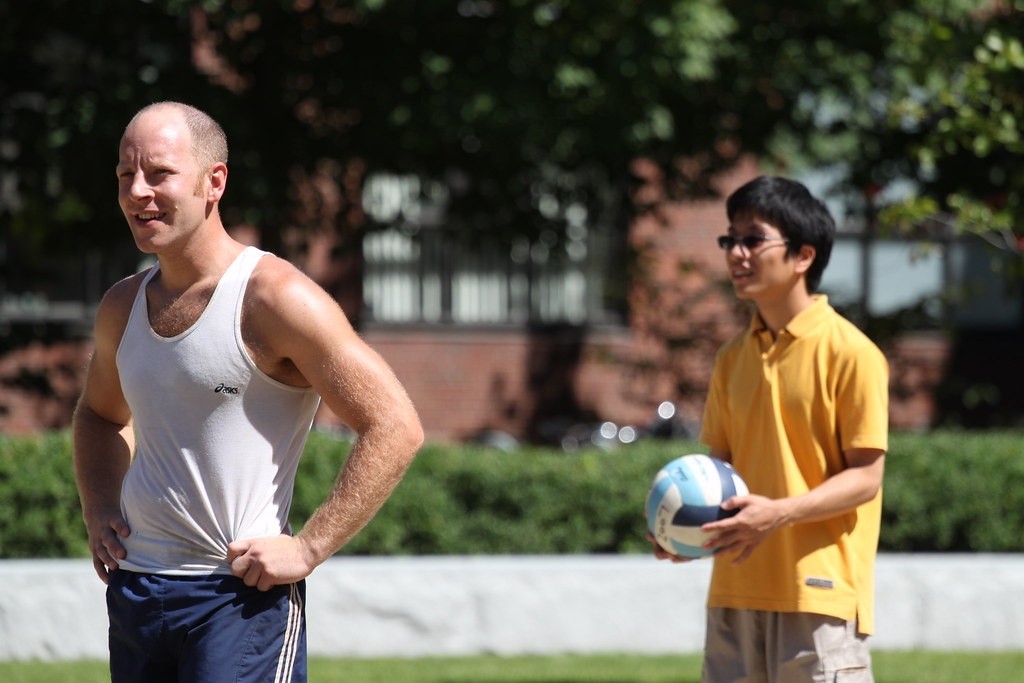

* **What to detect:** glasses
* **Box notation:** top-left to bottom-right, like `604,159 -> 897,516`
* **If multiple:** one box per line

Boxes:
716,236 -> 790,249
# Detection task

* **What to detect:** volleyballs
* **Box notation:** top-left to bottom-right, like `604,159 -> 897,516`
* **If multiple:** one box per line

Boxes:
643,453 -> 751,559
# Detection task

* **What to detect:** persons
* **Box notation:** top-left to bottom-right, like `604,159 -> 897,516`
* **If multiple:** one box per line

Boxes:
72,101 -> 424,683
643,174 -> 890,682
